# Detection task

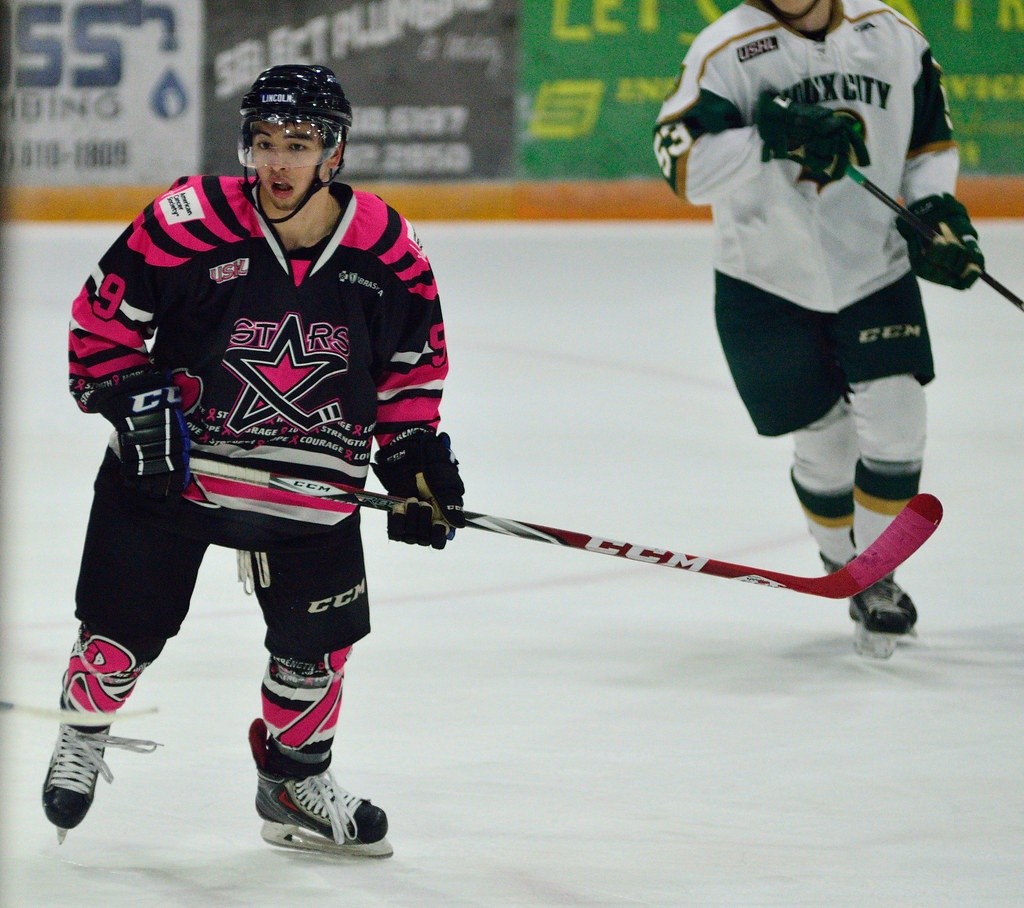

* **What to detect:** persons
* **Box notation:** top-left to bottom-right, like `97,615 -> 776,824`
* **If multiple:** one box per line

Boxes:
651,0 -> 986,660
43,64 -> 467,859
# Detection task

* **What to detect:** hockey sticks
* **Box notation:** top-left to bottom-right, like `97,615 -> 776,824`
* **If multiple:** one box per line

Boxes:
846,164 -> 1023,312
187,457 -> 944,600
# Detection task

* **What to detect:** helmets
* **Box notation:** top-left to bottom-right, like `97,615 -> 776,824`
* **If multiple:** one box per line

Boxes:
236,64 -> 352,132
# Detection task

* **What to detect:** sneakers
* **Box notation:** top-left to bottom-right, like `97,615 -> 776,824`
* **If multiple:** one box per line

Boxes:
41,724 -> 165,845
249,717 -> 394,859
819,550 -> 917,658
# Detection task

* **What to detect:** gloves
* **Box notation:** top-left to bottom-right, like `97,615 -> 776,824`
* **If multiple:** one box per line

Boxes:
369,426 -> 466,550
757,89 -> 870,180
86,374 -> 194,504
895,193 -> 984,290
